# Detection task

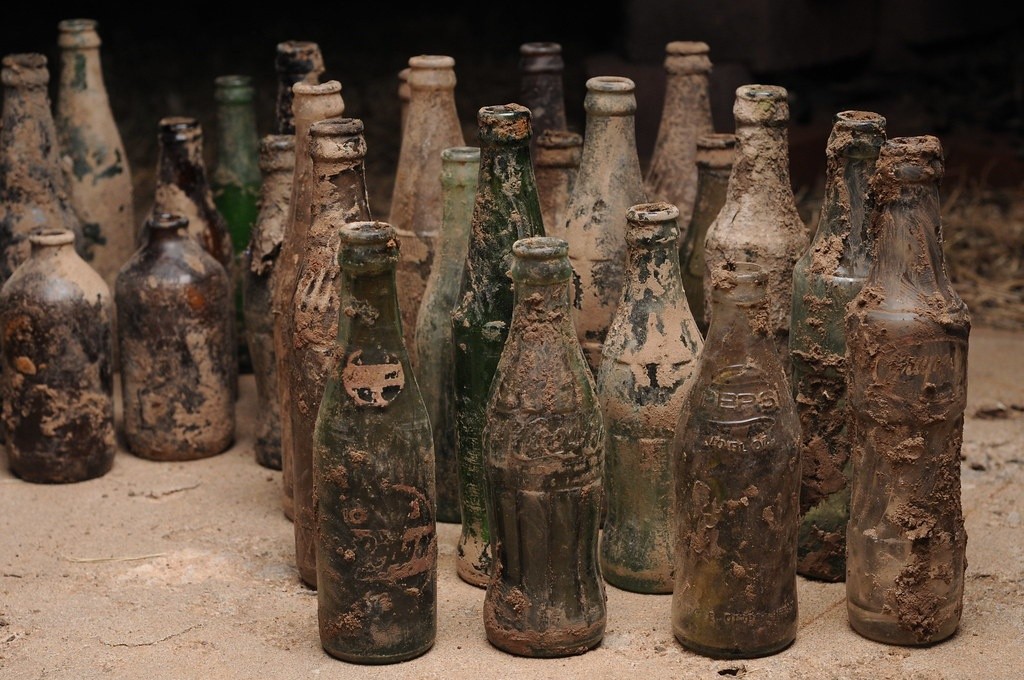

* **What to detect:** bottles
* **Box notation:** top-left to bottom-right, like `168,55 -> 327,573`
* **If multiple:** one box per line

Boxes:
0,13 -> 972,668
